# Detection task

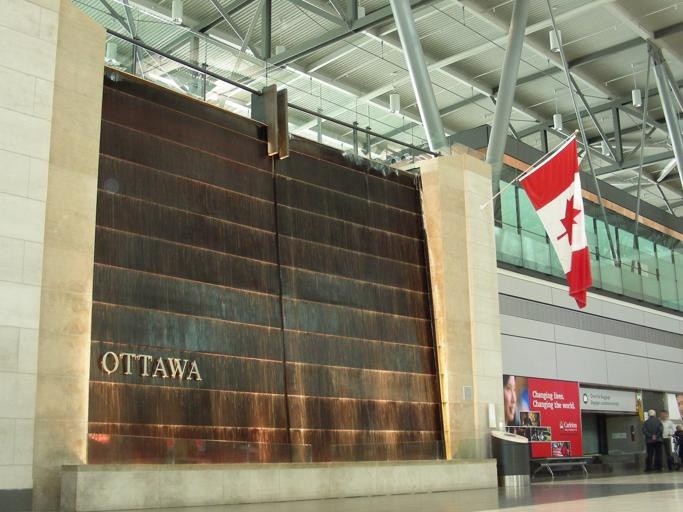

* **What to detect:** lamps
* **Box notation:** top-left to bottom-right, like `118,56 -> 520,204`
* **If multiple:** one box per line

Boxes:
632,64 -> 643,107
550,6 -> 563,53
553,89 -> 562,131
276,20 -> 286,70
390,86 -> 401,114
602,119 -> 610,156
106,19 -> 117,65
171,1 -> 184,25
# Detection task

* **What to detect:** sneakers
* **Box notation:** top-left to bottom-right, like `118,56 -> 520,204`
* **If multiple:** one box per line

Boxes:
645,467 -> 665,472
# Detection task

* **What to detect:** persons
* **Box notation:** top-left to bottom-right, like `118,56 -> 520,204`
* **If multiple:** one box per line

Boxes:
641,409 -> 664,474
658,409 -> 678,472
561,444 -> 568,456
503,375 -> 520,425
538,432 -> 545,440
674,423 -> 683,463
531,433 -> 535,440
524,412 -> 532,426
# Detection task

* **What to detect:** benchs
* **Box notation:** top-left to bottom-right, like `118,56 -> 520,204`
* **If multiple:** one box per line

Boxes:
531,455 -> 593,480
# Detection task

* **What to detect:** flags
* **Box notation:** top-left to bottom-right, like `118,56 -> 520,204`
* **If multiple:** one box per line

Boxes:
520,138 -> 595,309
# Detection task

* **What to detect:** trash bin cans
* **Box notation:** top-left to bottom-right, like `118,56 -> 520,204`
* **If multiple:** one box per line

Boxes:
491,431 -> 531,487
